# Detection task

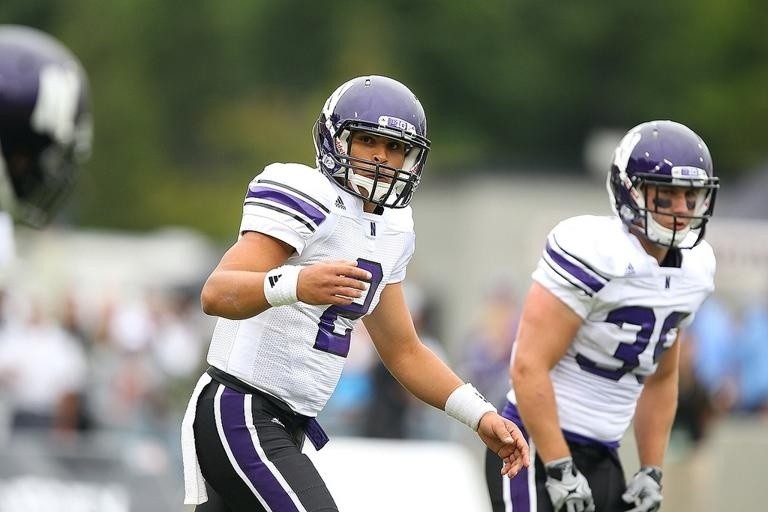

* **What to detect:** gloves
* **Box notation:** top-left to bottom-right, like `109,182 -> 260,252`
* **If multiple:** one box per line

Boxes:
543,456 -> 596,512
621,466 -> 666,512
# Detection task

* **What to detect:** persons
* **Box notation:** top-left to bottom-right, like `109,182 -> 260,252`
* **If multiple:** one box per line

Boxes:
182,84 -> 531,512
483,117 -> 722,510
0,22 -> 99,234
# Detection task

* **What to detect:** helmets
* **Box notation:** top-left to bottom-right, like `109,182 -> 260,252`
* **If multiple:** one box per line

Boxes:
1,24 -> 93,210
605,119 -> 714,232
316,74 -> 430,196
